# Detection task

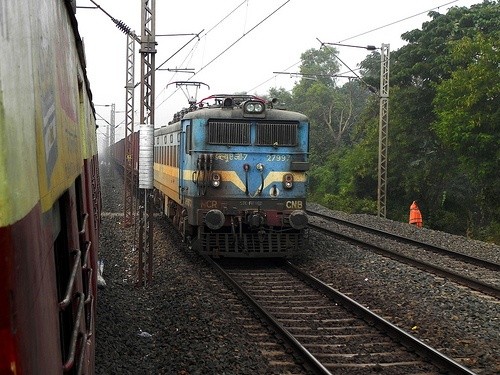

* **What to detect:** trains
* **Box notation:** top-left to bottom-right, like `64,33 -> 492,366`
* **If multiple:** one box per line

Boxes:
111,94 -> 310,263
0,0 -> 101,374
